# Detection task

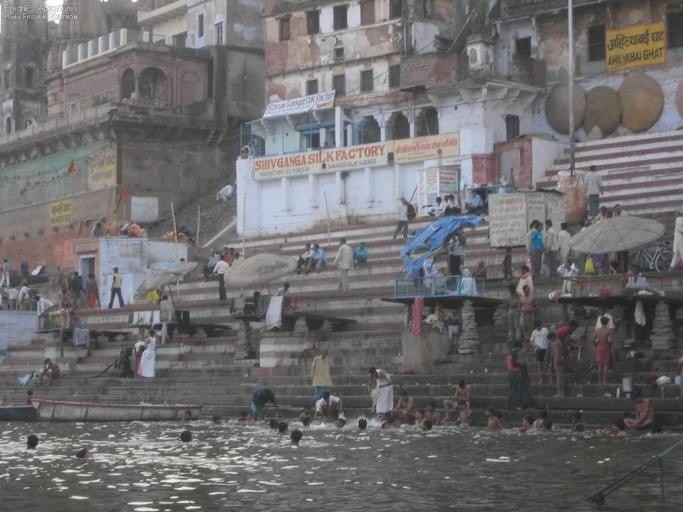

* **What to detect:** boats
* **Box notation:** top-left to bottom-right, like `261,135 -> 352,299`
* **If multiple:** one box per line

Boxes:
30,398 -> 203,422
0,404 -> 34,420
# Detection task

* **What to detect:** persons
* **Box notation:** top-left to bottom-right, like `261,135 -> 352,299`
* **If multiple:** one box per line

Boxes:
216,179 -> 233,202
1,220 -> 373,461
584,165 -> 604,212
357,189 -> 681,439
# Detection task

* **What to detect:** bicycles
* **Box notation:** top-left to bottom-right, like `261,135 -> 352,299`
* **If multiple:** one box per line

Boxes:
630,242 -> 674,273
548,347 -> 594,374
